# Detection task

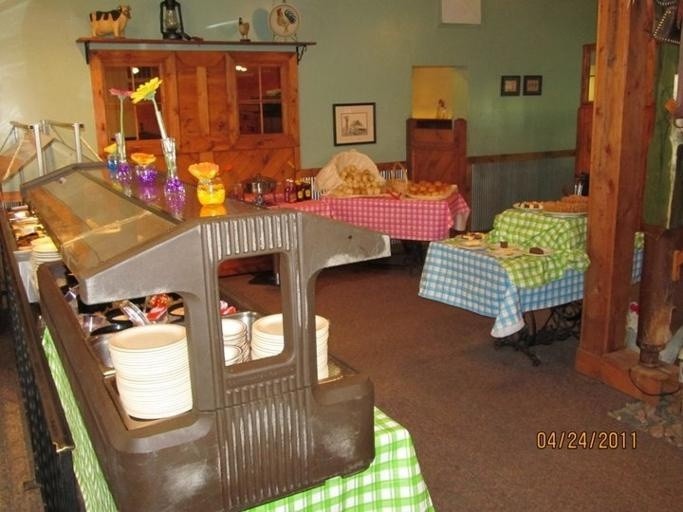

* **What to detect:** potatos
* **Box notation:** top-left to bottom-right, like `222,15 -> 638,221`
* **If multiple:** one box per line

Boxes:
409,180 -> 449,197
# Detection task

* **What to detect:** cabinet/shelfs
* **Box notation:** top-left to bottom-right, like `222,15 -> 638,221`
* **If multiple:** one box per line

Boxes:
89,49 -> 300,186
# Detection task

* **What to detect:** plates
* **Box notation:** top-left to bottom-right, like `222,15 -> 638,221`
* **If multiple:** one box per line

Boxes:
489,245 -> 550,258
510,200 -> 588,218
454,232 -> 488,250
109,324 -> 194,419
30,236 -> 61,289
250,313 -> 329,380
268,3 -> 301,37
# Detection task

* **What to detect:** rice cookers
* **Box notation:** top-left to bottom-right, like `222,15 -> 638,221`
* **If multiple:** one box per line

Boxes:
575,172 -> 589,196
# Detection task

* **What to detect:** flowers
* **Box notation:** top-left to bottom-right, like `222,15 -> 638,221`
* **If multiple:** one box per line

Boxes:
189,162 -> 220,179
131,153 -> 156,168
131,76 -> 175,177
105,143 -> 118,154
110,89 -> 131,161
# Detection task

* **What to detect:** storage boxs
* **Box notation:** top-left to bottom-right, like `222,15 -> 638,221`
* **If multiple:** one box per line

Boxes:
410,64 -> 468,120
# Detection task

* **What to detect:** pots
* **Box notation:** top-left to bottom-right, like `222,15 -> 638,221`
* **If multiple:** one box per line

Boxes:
241,175 -> 275,193
84,321 -> 134,369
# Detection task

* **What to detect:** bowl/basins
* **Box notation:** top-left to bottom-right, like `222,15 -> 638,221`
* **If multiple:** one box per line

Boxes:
220,318 -> 248,366
167,301 -> 184,321
104,306 -> 152,323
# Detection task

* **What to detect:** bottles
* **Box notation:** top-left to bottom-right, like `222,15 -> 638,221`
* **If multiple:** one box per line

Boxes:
285,177 -> 311,203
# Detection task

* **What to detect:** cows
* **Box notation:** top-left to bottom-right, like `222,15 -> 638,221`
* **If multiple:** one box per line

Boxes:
89,5 -> 131,37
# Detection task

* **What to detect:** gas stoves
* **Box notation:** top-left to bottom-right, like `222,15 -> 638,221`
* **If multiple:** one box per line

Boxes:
241,192 -> 277,206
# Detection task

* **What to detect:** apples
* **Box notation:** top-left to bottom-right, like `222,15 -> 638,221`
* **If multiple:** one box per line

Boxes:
332,164 -> 386,195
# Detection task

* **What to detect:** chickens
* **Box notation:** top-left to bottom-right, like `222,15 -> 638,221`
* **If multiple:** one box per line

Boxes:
237,17 -> 248,39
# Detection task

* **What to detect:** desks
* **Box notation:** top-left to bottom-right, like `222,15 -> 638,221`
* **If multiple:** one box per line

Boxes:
417,208 -> 645,369
262,199 -> 471,240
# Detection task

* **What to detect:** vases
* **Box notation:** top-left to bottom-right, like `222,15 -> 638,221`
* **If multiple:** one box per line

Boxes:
137,168 -> 157,183
195,178 -> 226,206
160,137 -> 188,211
117,162 -> 132,181
108,155 -> 119,170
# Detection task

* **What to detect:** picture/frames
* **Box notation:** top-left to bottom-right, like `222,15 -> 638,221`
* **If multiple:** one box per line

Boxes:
333,103 -> 377,146
524,75 -> 542,95
501,75 -> 521,96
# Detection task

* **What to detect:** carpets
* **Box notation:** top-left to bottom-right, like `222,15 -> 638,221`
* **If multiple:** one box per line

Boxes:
608,390 -> 683,447
